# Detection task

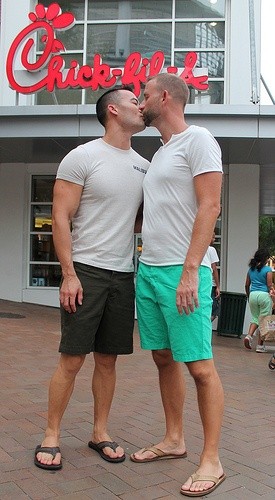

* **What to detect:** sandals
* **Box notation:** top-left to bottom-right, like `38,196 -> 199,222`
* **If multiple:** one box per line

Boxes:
269,355 -> 275,370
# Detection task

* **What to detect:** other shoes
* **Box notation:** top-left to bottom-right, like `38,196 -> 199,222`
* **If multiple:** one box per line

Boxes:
244,336 -> 252,349
255,345 -> 268,353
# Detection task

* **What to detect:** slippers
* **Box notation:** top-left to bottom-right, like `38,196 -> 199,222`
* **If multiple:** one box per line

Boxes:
33,444 -> 62,470
88,440 -> 126,463
130,446 -> 187,462
180,472 -> 227,497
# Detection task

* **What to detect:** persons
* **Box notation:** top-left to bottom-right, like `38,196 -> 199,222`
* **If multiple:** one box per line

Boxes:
130,73 -> 227,497
34,84 -> 151,471
207,230 -> 275,371
